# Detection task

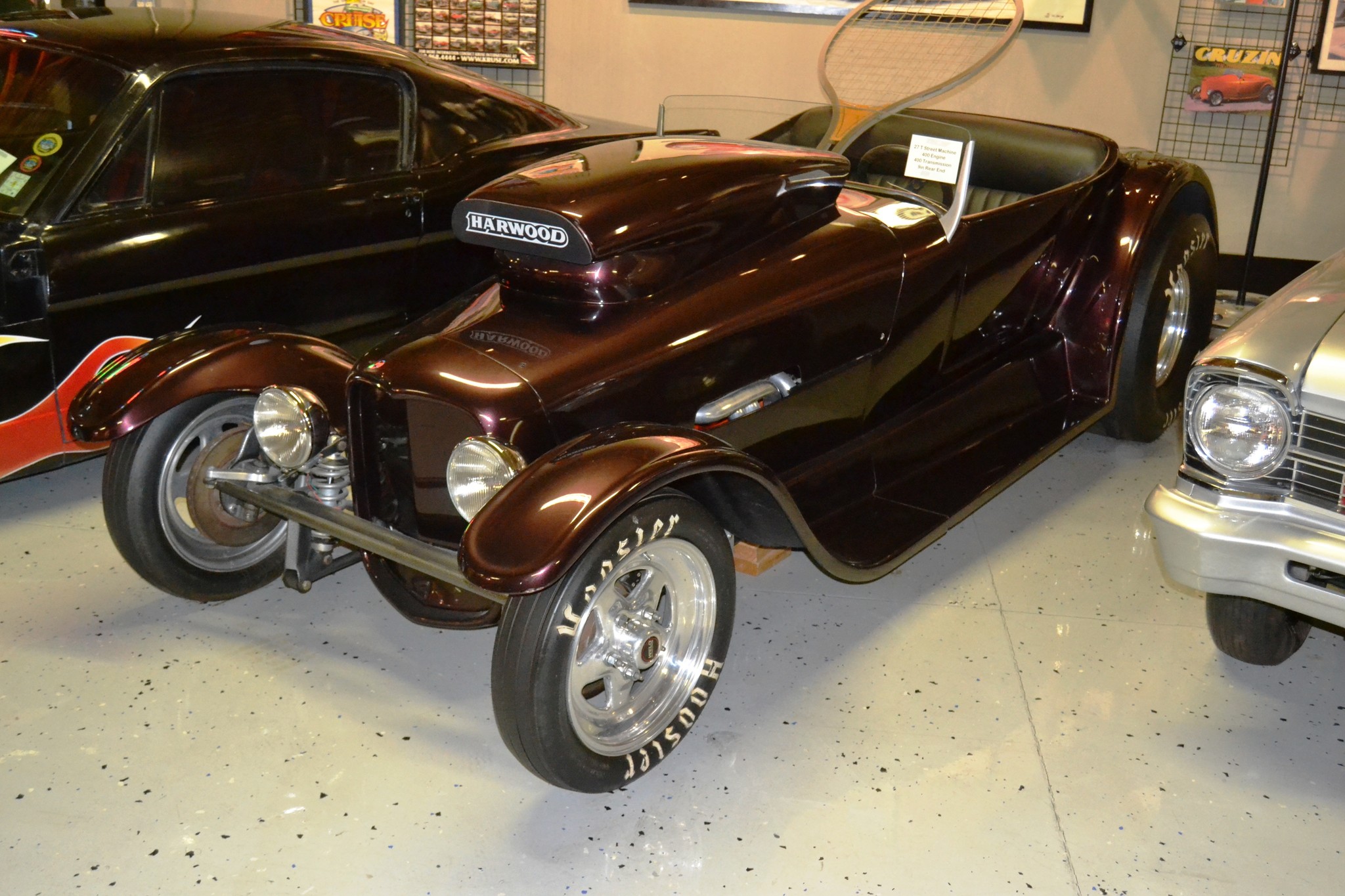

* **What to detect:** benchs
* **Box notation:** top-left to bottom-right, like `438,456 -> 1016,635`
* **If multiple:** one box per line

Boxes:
789,106 -> 1108,217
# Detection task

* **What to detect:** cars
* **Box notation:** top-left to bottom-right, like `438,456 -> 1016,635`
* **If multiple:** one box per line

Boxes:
1137,242 -> 1345,667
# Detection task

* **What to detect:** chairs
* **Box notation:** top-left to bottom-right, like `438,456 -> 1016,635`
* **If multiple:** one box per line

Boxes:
30,55 -> 124,133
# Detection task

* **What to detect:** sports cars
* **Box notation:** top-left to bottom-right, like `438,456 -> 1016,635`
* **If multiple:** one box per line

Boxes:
61,98 -> 1224,795
1190,70 -> 1277,107
0,8 -> 663,497
